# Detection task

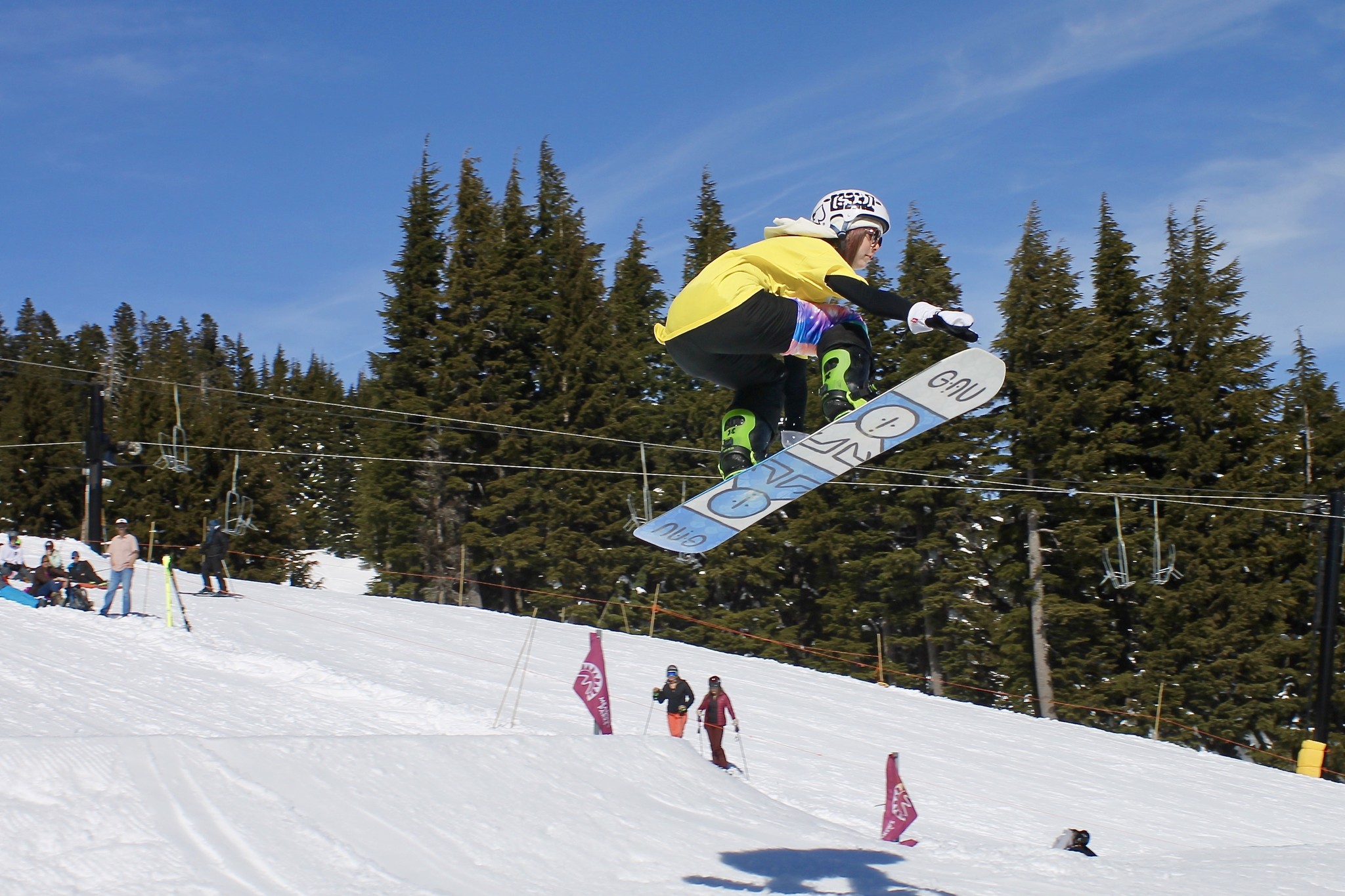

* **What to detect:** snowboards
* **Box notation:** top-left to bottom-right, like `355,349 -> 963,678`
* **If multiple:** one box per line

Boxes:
69,582 -> 123,589
632,346 -> 1007,554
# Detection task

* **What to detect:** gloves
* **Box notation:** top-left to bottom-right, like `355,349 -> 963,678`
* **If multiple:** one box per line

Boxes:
908,302 -> 978,343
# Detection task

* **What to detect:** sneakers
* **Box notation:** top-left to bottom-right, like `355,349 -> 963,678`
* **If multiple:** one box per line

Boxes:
213,591 -> 229,596
199,587 -> 213,593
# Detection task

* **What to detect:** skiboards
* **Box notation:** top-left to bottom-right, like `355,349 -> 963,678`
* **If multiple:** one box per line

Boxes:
178,591 -> 235,597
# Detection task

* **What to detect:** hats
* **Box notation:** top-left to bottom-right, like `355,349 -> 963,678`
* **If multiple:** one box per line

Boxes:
116,519 -> 128,523
209,519 -> 219,530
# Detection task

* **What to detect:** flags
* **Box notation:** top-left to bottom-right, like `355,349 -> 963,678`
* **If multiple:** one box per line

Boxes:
882,755 -> 917,847
573,632 -> 612,735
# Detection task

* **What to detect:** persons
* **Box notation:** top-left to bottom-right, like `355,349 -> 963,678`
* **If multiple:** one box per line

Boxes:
656,189 -> 979,480
95,519 -> 140,617
196,520 -> 228,596
653,665 -> 694,738
1063,829 -> 1097,857
697,676 -> 737,769
0,531 -> 107,612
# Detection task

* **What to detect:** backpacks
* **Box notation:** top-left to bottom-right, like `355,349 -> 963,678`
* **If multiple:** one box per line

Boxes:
66,588 -> 94,611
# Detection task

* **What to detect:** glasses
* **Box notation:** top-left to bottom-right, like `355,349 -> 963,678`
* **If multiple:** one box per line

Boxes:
865,229 -> 882,247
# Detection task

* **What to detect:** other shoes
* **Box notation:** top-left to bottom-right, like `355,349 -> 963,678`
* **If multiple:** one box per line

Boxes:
817,323 -> 885,422
717,405 -> 779,481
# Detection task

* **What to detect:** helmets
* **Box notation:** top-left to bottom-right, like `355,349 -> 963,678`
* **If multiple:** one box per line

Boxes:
1071,828 -> 1090,844
709,676 -> 720,687
667,665 -> 678,673
811,190 -> 890,235
